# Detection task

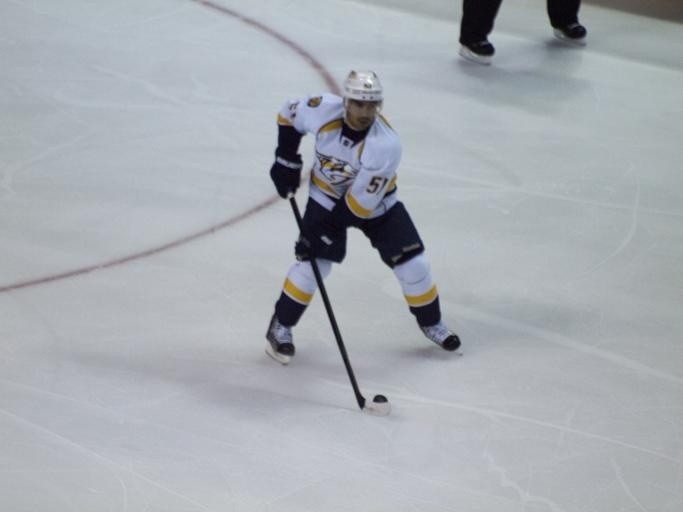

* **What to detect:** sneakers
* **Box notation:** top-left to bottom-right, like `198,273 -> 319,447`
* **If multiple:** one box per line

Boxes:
266,315 -> 295,356
459,35 -> 495,56
420,319 -> 461,352
552,19 -> 587,39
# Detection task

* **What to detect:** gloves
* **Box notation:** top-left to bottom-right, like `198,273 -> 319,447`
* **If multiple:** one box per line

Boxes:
270,148 -> 303,200
296,224 -> 340,256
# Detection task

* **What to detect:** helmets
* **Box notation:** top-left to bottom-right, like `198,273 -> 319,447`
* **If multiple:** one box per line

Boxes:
342,70 -> 383,104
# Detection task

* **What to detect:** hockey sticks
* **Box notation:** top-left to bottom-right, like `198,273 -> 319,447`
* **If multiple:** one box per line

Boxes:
288,191 -> 391,414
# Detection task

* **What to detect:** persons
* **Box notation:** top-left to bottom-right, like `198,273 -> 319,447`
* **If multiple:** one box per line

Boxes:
266,70 -> 463,355
458,1 -> 585,55
